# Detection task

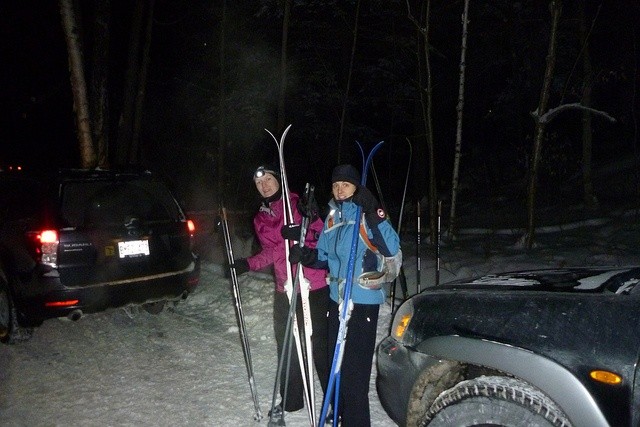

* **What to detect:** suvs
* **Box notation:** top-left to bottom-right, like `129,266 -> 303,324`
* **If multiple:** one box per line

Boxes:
0,161 -> 201,346
375,261 -> 640,427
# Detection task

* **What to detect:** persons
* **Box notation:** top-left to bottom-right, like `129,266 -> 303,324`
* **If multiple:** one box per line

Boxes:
225,162 -> 342,425
289,165 -> 403,427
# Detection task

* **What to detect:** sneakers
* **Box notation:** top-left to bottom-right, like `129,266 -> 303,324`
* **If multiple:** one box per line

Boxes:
326,410 -> 342,424
267,404 -> 303,419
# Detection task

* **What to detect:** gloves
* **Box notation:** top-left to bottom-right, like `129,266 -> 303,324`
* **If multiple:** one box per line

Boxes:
225,258 -> 249,278
361,250 -> 379,272
288,244 -> 311,264
281,223 -> 307,241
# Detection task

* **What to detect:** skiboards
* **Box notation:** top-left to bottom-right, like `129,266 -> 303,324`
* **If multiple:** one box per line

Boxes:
318,136 -> 384,423
263,118 -> 316,422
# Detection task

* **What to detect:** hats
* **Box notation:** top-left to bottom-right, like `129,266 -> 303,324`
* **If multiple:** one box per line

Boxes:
250,163 -> 282,182
330,164 -> 361,187
